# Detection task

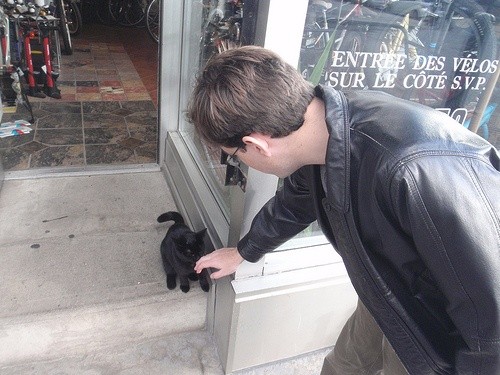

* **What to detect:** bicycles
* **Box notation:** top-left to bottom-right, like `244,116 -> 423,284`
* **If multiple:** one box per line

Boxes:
96,0 -> 121,27
126,1 -> 157,26
145,0 -> 159,42
57,1 -> 82,55
299,0 -> 500,149
109,0 -> 146,25
200,1 -> 245,57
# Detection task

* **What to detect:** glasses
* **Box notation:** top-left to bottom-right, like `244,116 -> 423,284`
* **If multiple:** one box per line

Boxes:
226,144 -> 244,167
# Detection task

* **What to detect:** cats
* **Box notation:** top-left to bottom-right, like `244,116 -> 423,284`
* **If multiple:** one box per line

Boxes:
157,211 -> 209,293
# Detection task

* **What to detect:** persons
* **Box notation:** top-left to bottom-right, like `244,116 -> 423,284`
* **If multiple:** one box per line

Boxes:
182,45 -> 500,375
